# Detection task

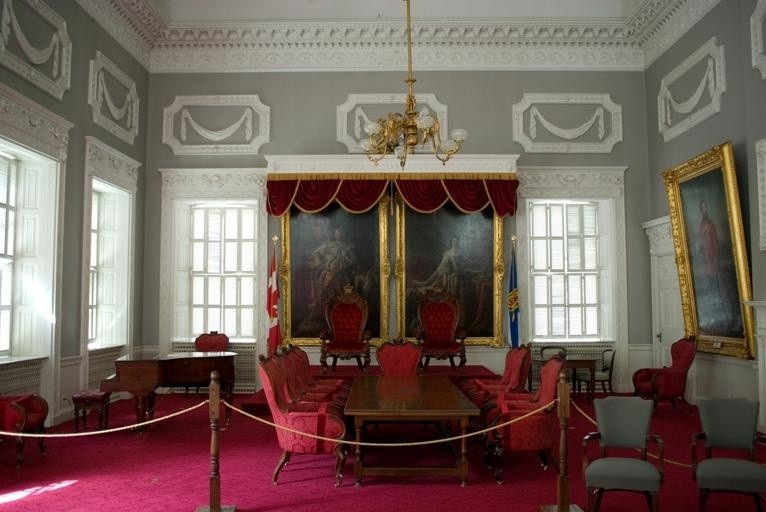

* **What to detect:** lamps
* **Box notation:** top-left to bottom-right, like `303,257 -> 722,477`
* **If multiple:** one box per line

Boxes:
361,0 -> 468,167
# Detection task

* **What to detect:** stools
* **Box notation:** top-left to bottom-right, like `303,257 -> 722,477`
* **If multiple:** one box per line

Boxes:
73,389 -> 112,432
0,394 -> 50,470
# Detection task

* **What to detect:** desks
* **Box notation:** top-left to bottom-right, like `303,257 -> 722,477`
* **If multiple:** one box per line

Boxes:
528,356 -> 596,395
345,360 -> 480,486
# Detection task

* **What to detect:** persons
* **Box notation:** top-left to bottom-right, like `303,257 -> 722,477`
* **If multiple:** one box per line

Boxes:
414,236 -> 480,296
308,226 -> 366,312
697,200 -> 736,337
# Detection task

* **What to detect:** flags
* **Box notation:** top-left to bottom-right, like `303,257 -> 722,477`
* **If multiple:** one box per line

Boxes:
265,235 -> 282,357
508,234 -> 520,349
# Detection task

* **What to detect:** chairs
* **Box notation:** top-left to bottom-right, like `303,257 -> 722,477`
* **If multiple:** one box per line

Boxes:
577,349 -> 617,398
541,347 -> 567,358
319,283 -> 372,374
691,396 -> 765,512
375,339 -> 422,375
477,352 -> 565,485
288,343 -> 352,389
272,352 -> 345,408
415,288 -> 467,374
258,354 -> 349,487
282,347 -> 349,395
633,335 -> 698,413
470,342 -> 533,404
459,347 -> 520,390
185,331 -> 229,395
582,396 -> 665,512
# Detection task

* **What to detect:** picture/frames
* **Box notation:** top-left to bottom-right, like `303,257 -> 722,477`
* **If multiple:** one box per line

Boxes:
395,194 -> 506,349
663,140 -> 752,359
281,190 -> 390,349
